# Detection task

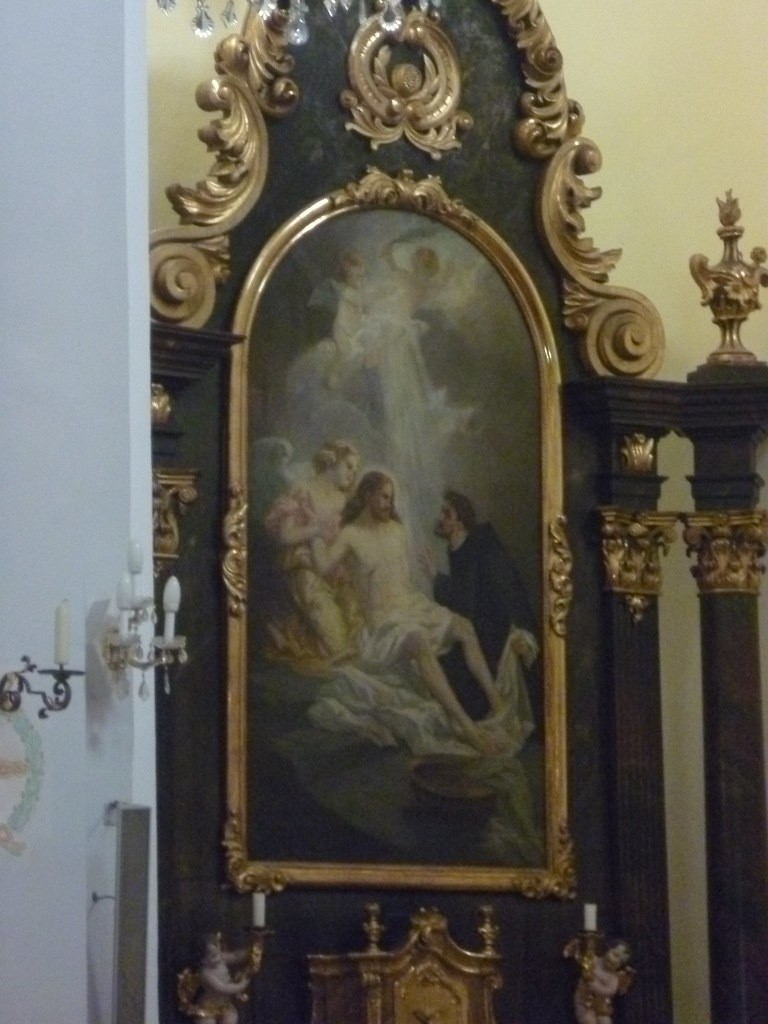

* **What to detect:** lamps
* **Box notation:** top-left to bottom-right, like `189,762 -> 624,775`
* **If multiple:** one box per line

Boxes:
101,540 -> 189,702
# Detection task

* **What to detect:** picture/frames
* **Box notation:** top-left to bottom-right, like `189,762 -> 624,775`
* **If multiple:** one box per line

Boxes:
222,171 -> 581,904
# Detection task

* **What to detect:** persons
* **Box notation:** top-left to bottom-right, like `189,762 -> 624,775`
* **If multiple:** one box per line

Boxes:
566,939 -> 632,1024
189,933 -> 243,1024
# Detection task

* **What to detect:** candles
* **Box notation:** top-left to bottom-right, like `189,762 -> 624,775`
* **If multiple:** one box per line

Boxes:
251,885 -> 265,929
583,903 -> 597,932
54,599 -> 71,666
